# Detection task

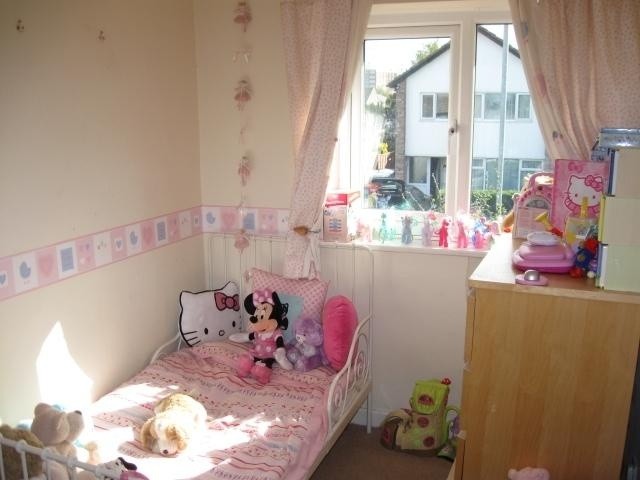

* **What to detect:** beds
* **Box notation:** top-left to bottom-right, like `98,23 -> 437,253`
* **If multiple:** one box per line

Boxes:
1,233 -> 376,480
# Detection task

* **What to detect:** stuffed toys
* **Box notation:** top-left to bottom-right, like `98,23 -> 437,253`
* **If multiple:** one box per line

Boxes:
233,287 -> 293,388
0,403 -> 149,480
270,310 -> 329,375
319,294 -> 357,371
177,274 -> 245,349
138,393 -> 209,460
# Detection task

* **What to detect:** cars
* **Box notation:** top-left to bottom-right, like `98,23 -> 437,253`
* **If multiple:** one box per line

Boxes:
365,176 -> 408,204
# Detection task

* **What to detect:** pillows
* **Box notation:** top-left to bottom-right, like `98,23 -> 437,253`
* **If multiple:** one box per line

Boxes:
176,267 -> 359,374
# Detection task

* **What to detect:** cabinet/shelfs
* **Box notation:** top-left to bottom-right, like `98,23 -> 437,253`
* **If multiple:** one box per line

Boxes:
448,226 -> 639,480
